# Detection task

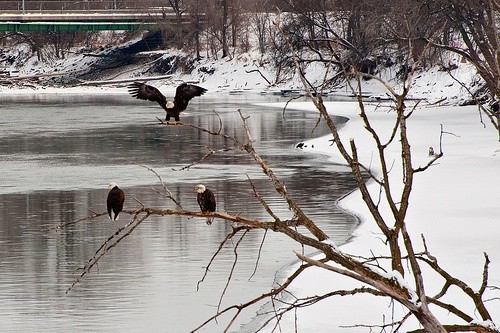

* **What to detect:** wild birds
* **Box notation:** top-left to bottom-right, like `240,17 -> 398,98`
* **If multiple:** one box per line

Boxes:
296,142 -> 304,149
